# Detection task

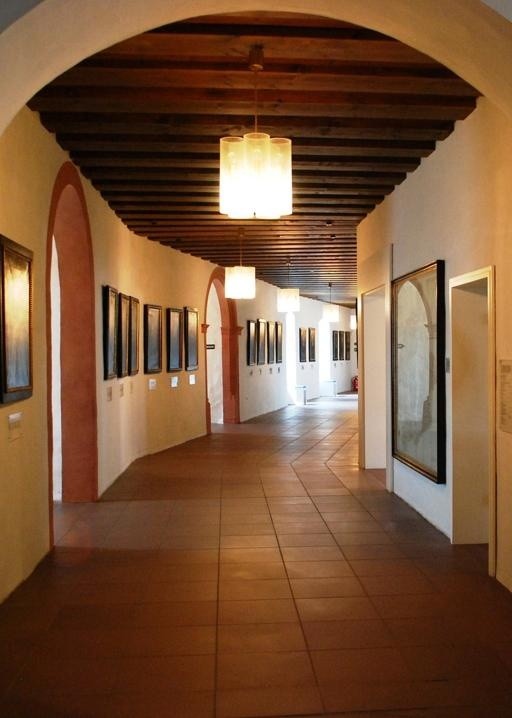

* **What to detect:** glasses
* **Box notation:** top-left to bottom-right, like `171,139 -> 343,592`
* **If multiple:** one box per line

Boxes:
215,43 -> 293,223
223,226 -> 339,324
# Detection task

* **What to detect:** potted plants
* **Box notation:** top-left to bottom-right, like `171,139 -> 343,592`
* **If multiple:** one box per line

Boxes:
0,233 -> 35,407
332,330 -> 352,362
299,327 -> 316,363
104,284 -> 200,381
246,317 -> 284,366
389,259 -> 446,485
